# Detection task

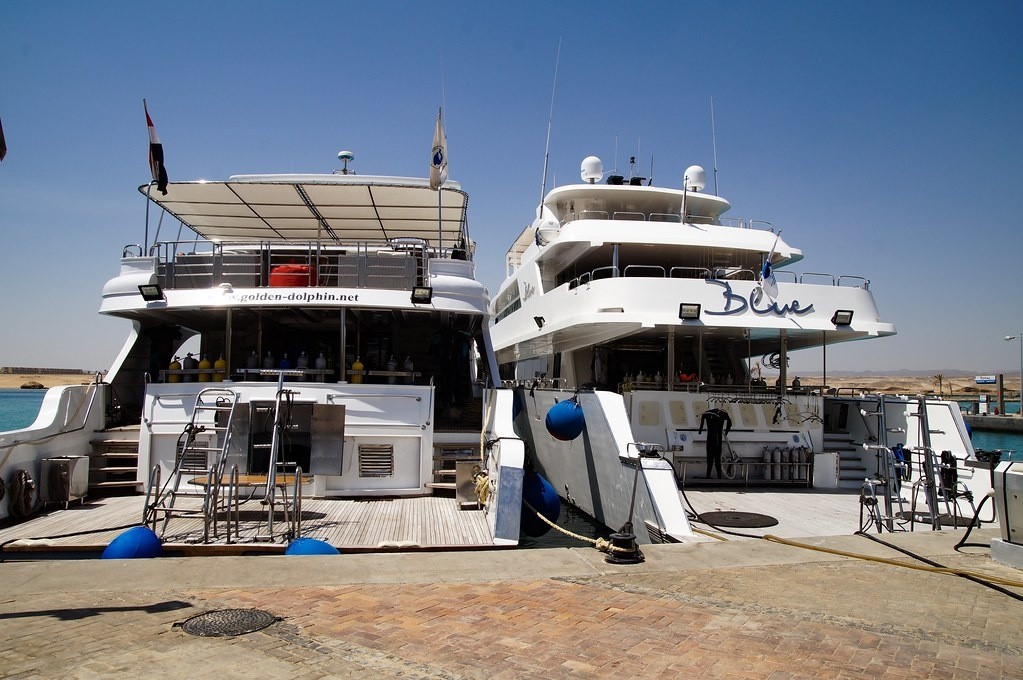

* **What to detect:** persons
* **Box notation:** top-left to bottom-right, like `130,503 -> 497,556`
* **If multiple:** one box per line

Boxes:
675,360 -> 696,381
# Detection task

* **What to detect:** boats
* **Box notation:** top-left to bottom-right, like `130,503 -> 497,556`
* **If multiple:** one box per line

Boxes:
472,35 -> 1023,546
0,90 -> 526,564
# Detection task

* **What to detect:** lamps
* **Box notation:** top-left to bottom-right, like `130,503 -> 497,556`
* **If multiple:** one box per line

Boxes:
138,284 -> 167,303
410,286 -> 435,309
534,316 -> 545,328
831,310 -> 854,326
679,303 -> 701,320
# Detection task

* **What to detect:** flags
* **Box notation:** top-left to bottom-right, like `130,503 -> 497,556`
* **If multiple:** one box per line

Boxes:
429,106 -> 447,192
761,240 -> 778,291
143,97 -> 168,196
0,120 -> 6,163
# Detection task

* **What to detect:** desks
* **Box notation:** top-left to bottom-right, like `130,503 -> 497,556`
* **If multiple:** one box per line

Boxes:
188,476 -> 315,520
